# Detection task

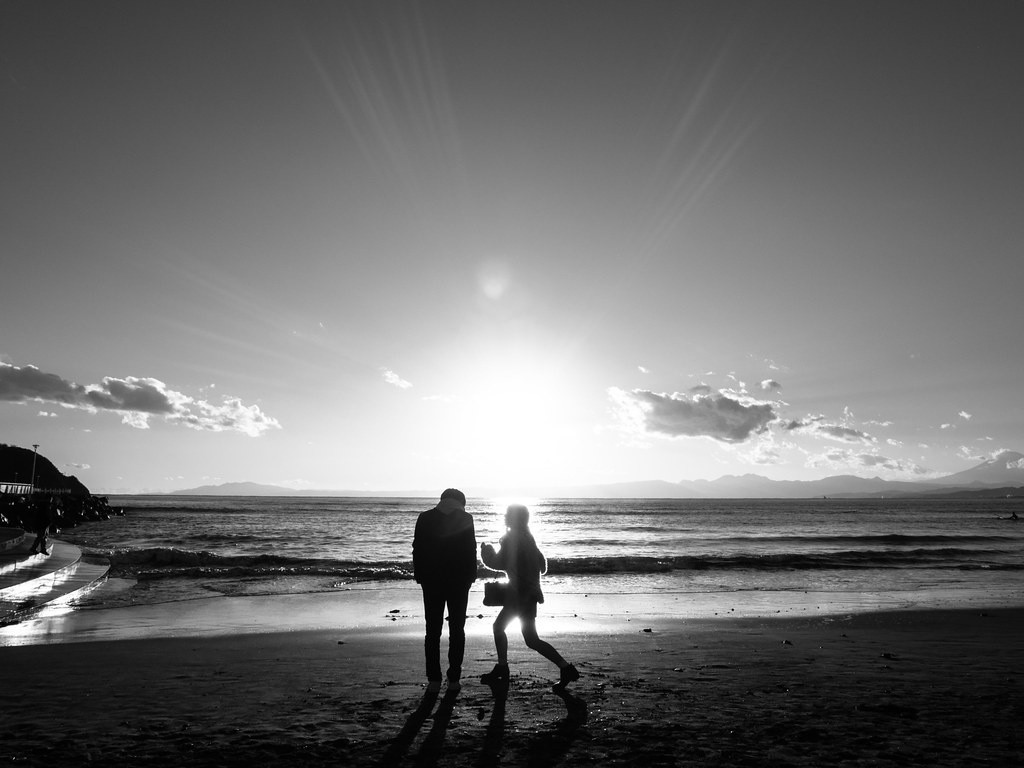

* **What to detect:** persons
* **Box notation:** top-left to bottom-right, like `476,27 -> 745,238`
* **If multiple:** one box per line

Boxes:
481,505 -> 580,692
31,492 -> 53,556
412,487 -> 478,692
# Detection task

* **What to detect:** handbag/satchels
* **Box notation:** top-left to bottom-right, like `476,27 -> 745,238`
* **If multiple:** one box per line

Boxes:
483,583 -> 511,606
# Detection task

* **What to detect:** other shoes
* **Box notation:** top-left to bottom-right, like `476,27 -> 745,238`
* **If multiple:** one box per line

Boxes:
426,665 -> 443,682
447,666 -> 461,682
552,662 -> 580,692
482,664 -> 510,679
